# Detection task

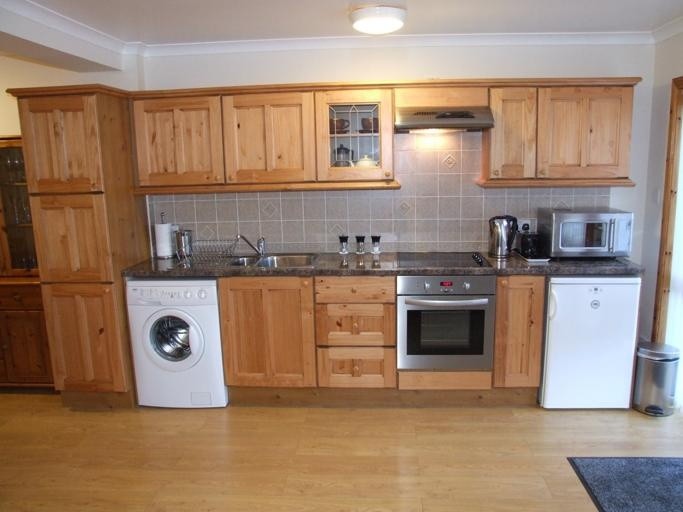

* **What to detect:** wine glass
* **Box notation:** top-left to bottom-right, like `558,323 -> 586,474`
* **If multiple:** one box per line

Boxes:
5,187 -> 30,223
3,149 -> 24,182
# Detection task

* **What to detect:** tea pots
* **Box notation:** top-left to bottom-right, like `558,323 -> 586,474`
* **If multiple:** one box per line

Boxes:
349,154 -> 380,168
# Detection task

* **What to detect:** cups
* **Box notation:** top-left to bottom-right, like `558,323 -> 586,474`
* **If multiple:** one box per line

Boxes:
329,118 -> 349,130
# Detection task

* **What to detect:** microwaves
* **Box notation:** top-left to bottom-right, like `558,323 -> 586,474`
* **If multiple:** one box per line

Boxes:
536,207 -> 634,258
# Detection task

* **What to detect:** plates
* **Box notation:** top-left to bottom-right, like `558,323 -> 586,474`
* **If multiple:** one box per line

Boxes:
330,130 -> 349,134
359,130 -> 378,133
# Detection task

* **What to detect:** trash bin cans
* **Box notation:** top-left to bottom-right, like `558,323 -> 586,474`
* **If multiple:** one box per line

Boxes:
632,342 -> 681,418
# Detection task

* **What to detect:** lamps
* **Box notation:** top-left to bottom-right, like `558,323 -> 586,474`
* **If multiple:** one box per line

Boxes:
347,4 -> 406,38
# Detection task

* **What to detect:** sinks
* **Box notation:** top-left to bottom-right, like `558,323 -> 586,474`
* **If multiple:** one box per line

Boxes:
227,257 -> 261,270
255,254 -> 318,270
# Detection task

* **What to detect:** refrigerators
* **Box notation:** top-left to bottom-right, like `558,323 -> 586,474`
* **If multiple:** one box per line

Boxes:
540,274 -> 641,412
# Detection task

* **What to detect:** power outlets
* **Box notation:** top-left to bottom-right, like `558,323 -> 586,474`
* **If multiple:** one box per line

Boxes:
517,218 -> 537,234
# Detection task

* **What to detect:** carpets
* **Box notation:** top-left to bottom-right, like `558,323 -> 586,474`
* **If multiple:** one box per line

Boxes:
567,453 -> 683,512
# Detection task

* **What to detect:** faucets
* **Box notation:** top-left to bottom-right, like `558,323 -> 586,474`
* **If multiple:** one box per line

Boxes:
236,233 -> 265,257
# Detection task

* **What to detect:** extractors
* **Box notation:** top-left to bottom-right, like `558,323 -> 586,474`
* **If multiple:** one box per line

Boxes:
393,106 -> 495,134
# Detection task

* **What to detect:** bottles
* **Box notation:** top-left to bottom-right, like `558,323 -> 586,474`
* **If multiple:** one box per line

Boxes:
356,255 -> 365,269
355,235 -> 365,254
340,254 -> 348,269
372,255 -> 380,268
370,235 -> 382,254
338,235 -> 349,254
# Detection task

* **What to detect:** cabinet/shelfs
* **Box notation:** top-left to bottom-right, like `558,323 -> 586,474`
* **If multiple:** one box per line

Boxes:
314,80 -> 402,193
492,275 -> 546,407
128,82 -> 317,196
537,75 -> 644,189
217,276 -> 316,411
5,82 -> 153,412
312,275 -> 401,410
0,135 -> 40,278
471,76 -> 536,189
0,280 -> 57,394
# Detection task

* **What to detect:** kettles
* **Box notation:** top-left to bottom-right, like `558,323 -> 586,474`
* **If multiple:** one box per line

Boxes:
488,215 -> 518,259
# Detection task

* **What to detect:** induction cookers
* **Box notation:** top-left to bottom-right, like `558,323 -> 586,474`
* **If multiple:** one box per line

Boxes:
398,252 -> 492,268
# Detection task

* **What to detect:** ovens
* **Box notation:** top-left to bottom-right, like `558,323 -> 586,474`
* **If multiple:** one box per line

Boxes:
395,295 -> 494,371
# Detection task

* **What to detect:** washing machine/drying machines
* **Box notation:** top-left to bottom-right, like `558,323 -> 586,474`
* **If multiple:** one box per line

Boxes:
124,278 -> 228,410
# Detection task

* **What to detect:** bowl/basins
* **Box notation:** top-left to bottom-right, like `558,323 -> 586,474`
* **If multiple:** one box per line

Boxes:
360,117 -> 378,130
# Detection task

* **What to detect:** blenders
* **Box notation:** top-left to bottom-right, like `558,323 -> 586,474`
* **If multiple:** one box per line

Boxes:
333,143 -> 353,167
